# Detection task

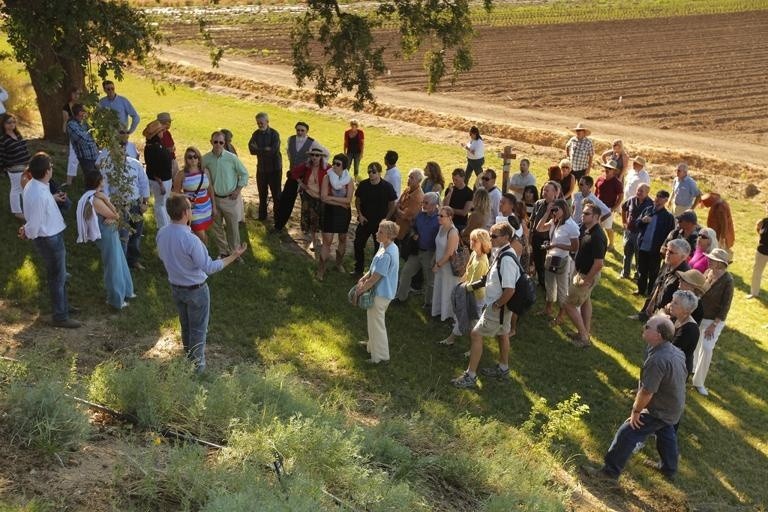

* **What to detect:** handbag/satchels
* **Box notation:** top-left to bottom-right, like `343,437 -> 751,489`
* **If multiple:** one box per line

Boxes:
543,253 -> 569,274
347,284 -> 373,309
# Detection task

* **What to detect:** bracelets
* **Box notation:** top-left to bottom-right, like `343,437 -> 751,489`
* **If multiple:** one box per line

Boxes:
632,407 -> 640,413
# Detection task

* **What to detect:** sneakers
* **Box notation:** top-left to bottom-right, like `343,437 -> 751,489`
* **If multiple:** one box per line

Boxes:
478,364 -> 510,380
103,293 -> 137,311
52,305 -> 82,329
256,217 -> 316,238
357,340 -> 391,365
617,273 -> 647,320
580,465 -> 617,488
642,460 -> 672,480
392,286 -> 592,359
130,263 -> 148,275
306,245 -> 364,282
449,372 -> 478,390
696,386 -> 709,396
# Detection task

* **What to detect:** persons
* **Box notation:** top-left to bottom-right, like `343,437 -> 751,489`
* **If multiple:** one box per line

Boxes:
156,196 -> 246,376
248,112 -> 402,281
580,310 -> 687,485
76,171 -> 138,312
0,114 -> 31,221
461,126 -> 484,184
355,221 -> 404,367
14,151 -> 82,331
0,82 -> 11,124
622,156 -> 736,396
61,80 -> 246,258
393,159 -> 539,357
746,214 -> 768,300
451,223 -> 522,388
531,122 -> 626,348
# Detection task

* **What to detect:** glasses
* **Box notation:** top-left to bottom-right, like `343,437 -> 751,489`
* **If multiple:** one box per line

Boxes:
551,206 -> 561,212
664,247 -> 680,254
186,154 -> 199,159
437,213 -> 449,217
212,139 -> 224,145
482,176 -> 494,181
333,161 -> 343,167
368,170 -> 379,174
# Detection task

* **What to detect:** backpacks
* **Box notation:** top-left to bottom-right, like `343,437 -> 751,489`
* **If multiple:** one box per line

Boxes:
496,251 -> 537,315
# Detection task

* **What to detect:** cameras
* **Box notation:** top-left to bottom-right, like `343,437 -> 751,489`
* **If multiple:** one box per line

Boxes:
539,240 -> 550,250
471,274 -> 487,291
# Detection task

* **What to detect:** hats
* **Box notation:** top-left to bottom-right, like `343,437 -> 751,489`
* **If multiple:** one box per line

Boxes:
675,269 -> 707,295
570,121 -> 722,224
702,247 -> 730,267
143,111 -> 328,157
